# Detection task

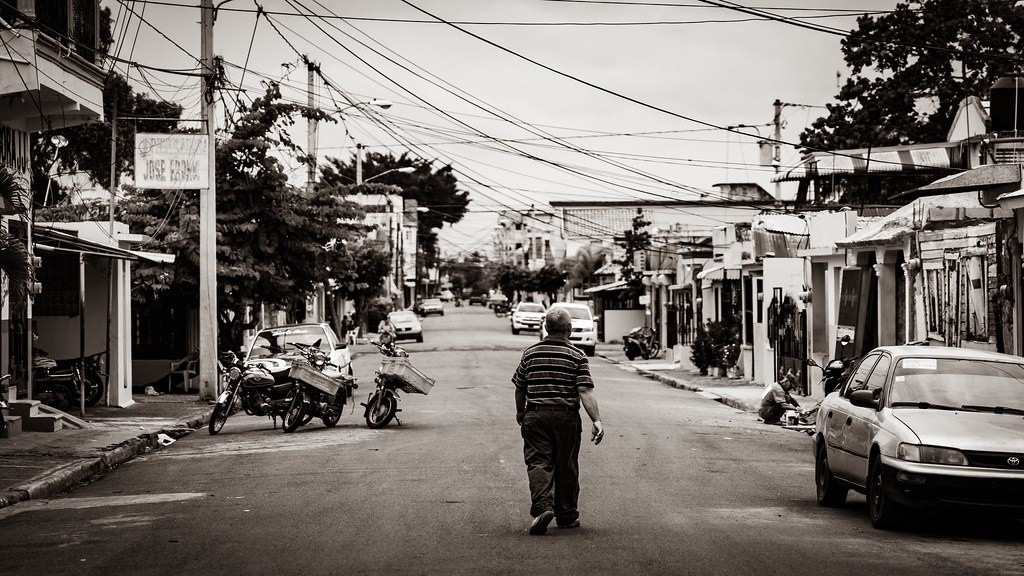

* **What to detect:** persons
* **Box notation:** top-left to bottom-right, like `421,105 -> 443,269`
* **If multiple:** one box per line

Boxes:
340,315 -> 355,338
758,373 -> 802,427
378,315 -> 397,345
511,307 -> 604,535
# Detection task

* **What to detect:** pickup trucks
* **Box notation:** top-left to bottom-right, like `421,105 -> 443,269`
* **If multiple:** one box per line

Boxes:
236,321 -> 354,397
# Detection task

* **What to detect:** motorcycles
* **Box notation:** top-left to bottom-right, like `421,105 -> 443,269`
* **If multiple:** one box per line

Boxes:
281,339 -> 360,433
208,349 -> 294,435
32,345 -> 108,413
360,340 -> 409,429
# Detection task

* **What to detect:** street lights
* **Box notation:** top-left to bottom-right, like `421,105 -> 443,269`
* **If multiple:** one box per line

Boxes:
307,99 -> 392,206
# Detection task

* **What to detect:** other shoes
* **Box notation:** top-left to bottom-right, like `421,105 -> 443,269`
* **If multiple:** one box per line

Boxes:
530,511 -> 555,535
558,519 -> 580,528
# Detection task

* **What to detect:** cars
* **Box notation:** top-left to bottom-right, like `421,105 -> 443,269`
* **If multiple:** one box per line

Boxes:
435,286 -> 511,318
384,309 -> 425,342
810,343 -> 1023,535
538,301 -> 601,356
510,301 -> 547,335
418,298 -> 444,318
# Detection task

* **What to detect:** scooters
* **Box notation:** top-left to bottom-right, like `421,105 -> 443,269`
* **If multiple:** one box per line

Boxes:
622,326 -> 660,361
805,334 -> 859,397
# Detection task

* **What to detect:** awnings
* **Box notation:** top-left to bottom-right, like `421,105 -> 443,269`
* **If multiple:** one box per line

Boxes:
7,217 -> 176,267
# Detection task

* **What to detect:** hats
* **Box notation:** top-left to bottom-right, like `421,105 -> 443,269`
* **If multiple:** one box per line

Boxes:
786,373 -> 797,391
